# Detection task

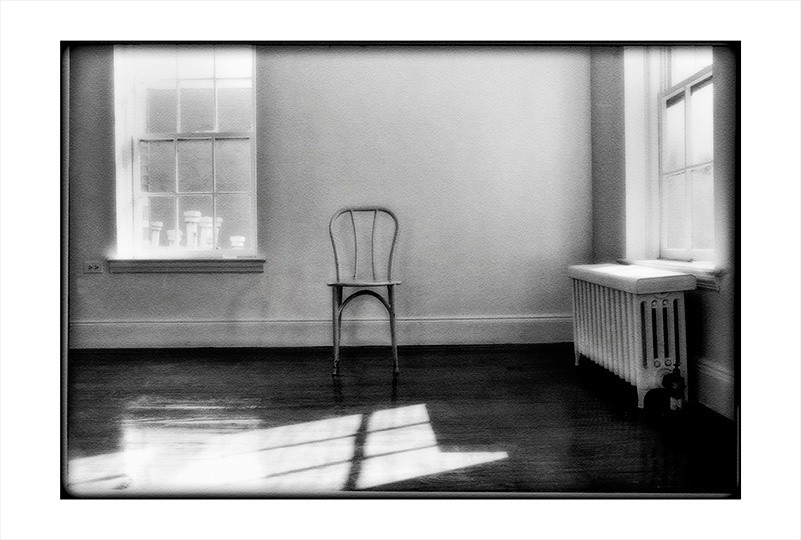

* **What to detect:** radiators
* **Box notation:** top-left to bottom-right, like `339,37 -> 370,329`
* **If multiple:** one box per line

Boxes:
568,262 -> 697,412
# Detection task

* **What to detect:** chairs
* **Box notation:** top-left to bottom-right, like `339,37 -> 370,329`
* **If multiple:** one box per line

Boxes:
326,204 -> 403,374
